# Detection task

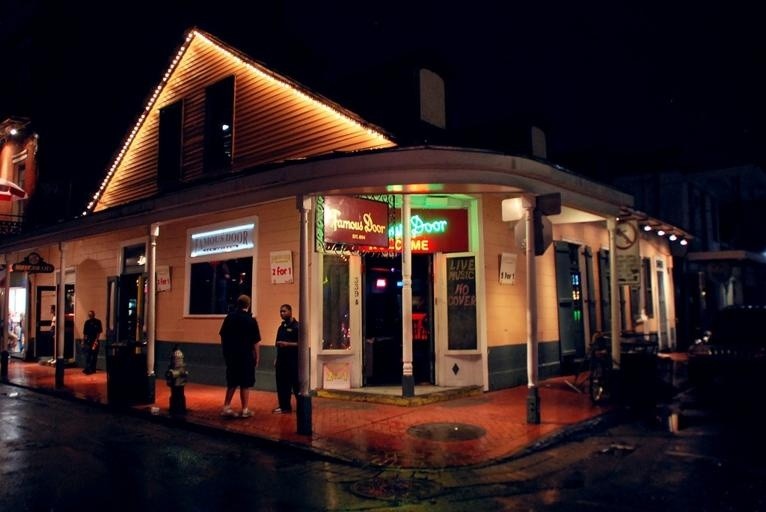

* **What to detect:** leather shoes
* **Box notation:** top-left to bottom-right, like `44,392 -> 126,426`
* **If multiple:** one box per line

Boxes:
272,407 -> 290,412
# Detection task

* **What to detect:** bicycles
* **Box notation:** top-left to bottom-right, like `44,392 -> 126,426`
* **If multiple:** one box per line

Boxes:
584,330 -> 612,407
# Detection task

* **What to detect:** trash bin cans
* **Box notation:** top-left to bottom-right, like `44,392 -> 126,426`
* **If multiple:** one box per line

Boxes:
103,340 -> 149,409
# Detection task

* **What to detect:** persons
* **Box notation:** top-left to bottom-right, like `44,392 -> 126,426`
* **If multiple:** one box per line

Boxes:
46,304 -> 56,366
7,312 -> 24,353
271,304 -> 299,413
81,310 -> 103,374
412,294 -> 430,381
217,293 -> 262,419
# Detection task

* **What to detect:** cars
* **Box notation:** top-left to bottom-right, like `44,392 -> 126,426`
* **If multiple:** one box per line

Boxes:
685,303 -> 763,401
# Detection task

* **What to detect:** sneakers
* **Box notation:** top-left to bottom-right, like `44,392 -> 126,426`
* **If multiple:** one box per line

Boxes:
220,406 -> 239,417
239,410 -> 255,418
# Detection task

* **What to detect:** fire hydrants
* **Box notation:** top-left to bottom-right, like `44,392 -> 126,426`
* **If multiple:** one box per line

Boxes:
163,348 -> 190,415
